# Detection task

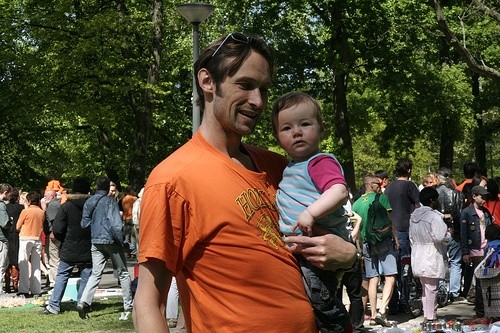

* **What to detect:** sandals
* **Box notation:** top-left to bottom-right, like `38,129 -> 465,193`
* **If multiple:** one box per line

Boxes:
374,312 -> 391,327
369,318 -> 377,326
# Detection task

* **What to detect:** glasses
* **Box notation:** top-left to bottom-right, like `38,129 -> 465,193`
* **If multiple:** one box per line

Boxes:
373,182 -> 380,186
211,33 -> 253,60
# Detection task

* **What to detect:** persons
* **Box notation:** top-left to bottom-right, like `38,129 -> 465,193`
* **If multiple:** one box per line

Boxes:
0,173 -> 178,327
272,92 -> 359,333
338,157 -> 500,325
132,31 -> 362,333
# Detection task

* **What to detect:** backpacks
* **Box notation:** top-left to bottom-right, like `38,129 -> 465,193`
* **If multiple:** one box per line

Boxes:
365,194 -> 393,245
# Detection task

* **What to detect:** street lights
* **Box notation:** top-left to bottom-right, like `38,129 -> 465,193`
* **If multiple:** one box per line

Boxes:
177,2 -> 219,140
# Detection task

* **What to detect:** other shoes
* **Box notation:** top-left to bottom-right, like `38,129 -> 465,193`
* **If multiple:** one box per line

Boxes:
76,303 -> 88,320
459,299 -> 473,305
399,308 -> 408,312
389,309 -> 396,314
16,292 -> 29,297
464,315 -> 487,325
38,310 -> 50,314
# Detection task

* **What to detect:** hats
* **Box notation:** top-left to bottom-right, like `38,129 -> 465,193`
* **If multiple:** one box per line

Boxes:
73,177 -> 90,195
471,185 -> 489,195
